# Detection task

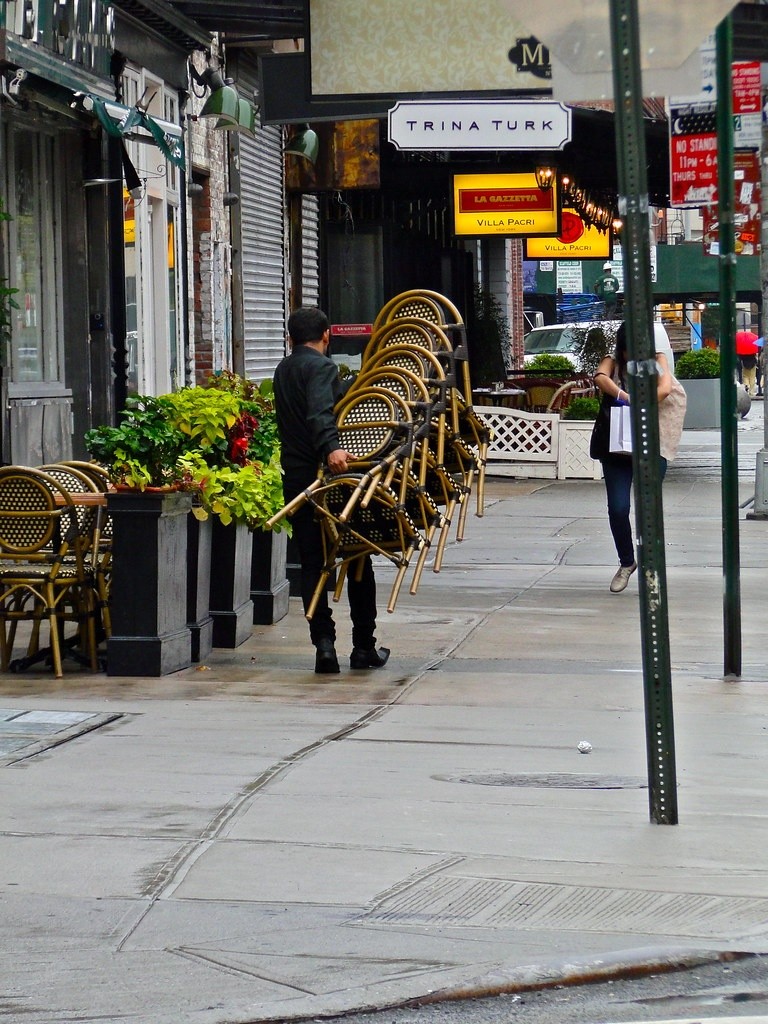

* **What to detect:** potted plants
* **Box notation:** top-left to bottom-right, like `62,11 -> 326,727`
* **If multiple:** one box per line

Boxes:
558,397 -> 605,480
84,371 -> 292,678
675,347 -> 723,429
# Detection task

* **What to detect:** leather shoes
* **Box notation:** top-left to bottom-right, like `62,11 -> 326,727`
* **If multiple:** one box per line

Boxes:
314,637 -> 341,674
609,559 -> 637,592
349,646 -> 391,669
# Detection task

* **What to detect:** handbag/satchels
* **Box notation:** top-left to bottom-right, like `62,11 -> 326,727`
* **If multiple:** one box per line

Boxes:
608,389 -> 633,456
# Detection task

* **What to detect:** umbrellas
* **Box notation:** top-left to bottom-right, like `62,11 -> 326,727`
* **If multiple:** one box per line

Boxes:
753,336 -> 764,346
737,330 -> 759,354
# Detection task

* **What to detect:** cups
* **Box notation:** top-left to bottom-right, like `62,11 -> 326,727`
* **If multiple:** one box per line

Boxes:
492,382 -> 499,391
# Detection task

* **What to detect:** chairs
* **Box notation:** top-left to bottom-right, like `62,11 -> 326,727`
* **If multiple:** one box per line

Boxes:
0,459 -> 113,677
265,288 -> 496,620
480,375 -> 599,452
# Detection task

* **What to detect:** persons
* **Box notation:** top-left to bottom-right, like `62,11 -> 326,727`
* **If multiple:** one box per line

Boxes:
738,346 -> 765,395
594,263 -> 619,319
588,319 -> 671,594
272,308 -> 391,673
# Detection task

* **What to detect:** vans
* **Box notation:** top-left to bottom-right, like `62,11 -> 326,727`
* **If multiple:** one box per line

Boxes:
521,321 -> 676,384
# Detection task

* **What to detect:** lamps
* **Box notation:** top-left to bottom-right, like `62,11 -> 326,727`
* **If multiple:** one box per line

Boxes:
282,122 -> 319,164
534,152 -> 622,237
190,47 -> 241,127
82,93 -> 184,138
212,58 -> 255,137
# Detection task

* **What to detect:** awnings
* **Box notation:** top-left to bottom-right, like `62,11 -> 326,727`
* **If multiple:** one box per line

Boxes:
16,82 -> 186,173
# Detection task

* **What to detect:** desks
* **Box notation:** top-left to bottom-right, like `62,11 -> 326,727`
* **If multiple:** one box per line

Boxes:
475,389 -> 526,405
570,387 -> 590,396
0,493 -> 109,670
